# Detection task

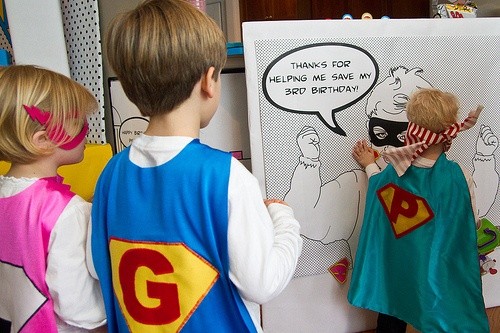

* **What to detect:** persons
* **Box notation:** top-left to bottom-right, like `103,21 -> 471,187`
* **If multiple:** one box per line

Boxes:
347,89 -> 492,333
0,66 -> 108,333
85,0 -> 303,333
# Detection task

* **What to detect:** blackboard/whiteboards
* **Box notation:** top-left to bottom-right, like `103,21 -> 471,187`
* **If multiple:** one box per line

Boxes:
241,16 -> 500,333
